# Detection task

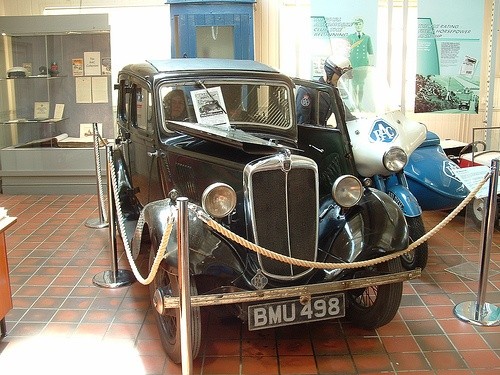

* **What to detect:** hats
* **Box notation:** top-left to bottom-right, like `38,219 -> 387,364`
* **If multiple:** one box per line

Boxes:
351,16 -> 366,24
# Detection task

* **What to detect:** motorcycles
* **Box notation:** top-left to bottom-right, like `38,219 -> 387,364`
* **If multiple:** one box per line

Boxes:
329,60 -> 500,273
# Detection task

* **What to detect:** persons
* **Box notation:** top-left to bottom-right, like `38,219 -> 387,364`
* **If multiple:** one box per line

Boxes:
163,88 -> 191,122
296,54 -> 356,127
345,17 -> 378,112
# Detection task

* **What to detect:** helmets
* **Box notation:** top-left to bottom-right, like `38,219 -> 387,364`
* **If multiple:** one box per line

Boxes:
324,54 -> 353,79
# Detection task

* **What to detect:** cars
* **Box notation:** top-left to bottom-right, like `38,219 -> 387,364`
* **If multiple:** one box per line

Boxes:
107,61 -> 421,366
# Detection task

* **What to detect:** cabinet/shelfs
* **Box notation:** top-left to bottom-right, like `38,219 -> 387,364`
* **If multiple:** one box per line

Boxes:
0,74 -> 70,125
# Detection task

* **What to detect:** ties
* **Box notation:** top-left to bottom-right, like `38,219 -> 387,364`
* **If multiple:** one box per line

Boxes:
358,33 -> 361,39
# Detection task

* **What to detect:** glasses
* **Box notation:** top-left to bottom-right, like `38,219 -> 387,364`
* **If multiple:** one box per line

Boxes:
335,66 -> 353,78
